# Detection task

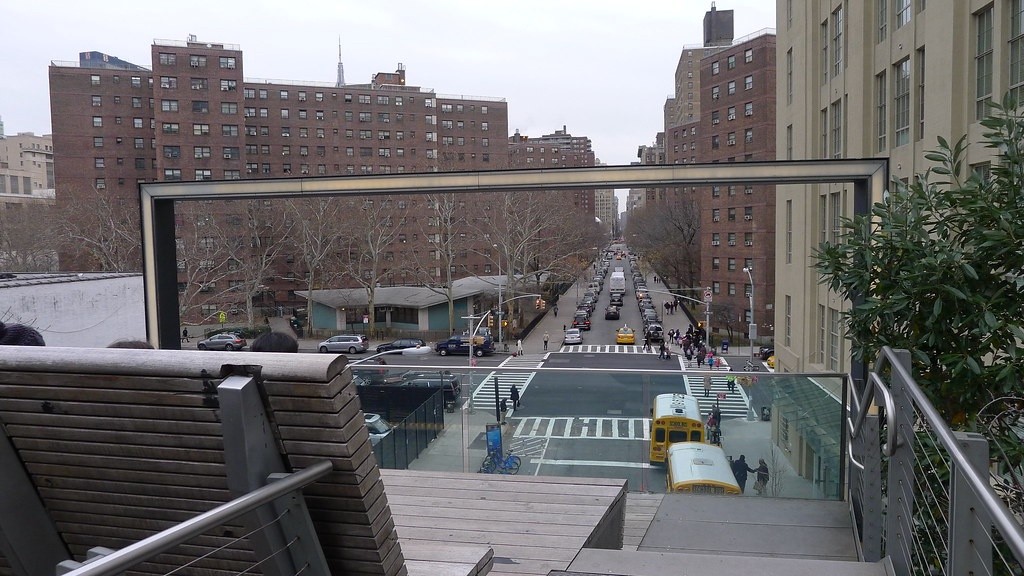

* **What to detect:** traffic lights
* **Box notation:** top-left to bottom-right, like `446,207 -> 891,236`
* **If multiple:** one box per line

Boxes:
536,299 -> 545,309
488,313 -> 494,327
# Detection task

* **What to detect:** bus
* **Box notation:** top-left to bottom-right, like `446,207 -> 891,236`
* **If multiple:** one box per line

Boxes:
665,442 -> 743,496
649,393 -> 704,467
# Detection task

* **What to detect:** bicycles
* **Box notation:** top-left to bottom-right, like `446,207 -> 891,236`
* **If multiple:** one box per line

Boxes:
480,446 -> 521,474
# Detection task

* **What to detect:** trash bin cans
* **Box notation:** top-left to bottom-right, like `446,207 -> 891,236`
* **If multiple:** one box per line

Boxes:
761,406 -> 771,422
711,346 -> 717,355
504,344 -> 510,352
446,404 -> 454,413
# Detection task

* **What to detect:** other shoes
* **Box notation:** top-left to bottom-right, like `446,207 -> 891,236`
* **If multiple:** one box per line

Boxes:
501,422 -> 507,425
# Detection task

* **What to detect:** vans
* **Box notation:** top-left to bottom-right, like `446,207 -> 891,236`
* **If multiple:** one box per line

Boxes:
435,334 -> 490,357
317,334 -> 368,354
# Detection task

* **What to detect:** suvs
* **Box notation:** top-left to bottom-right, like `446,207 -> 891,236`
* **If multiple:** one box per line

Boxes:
377,338 -> 425,352
384,370 -> 462,401
347,355 -> 388,386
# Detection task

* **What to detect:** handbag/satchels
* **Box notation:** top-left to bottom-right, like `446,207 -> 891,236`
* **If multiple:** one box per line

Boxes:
517,400 -> 520,407
752,477 -> 764,492
710,378 -> 713,384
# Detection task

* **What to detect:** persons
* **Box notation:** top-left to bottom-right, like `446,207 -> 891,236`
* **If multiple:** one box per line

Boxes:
451,329 -> 456,335
726,369 -> 736,394
734,455 -> 755,494
500,397 -> 508,425
653,276 -> 661,283
705,412 -> 714,440
181,327 -> 189,342
712,404 -> 720,425
554,305 -> 558,316
664,298 -> 678,315
1,320 -> 45,347
753,459 -> 769,496
703,376 -> 713,397
250,331 -> 298,354
553,302 -> 557,306
516,338 -> 523,356
510,384 -> 520,411
543,331 -> 549,351
642,324 -> 721,370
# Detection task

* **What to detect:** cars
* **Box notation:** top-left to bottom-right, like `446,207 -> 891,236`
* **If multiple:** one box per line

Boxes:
197,333 -> 247,351
616,325 -> 635,344
573,244 -> 664,341
363,411 -> 393,446
563,329 -> 583,344
759,345 -> 774,367
